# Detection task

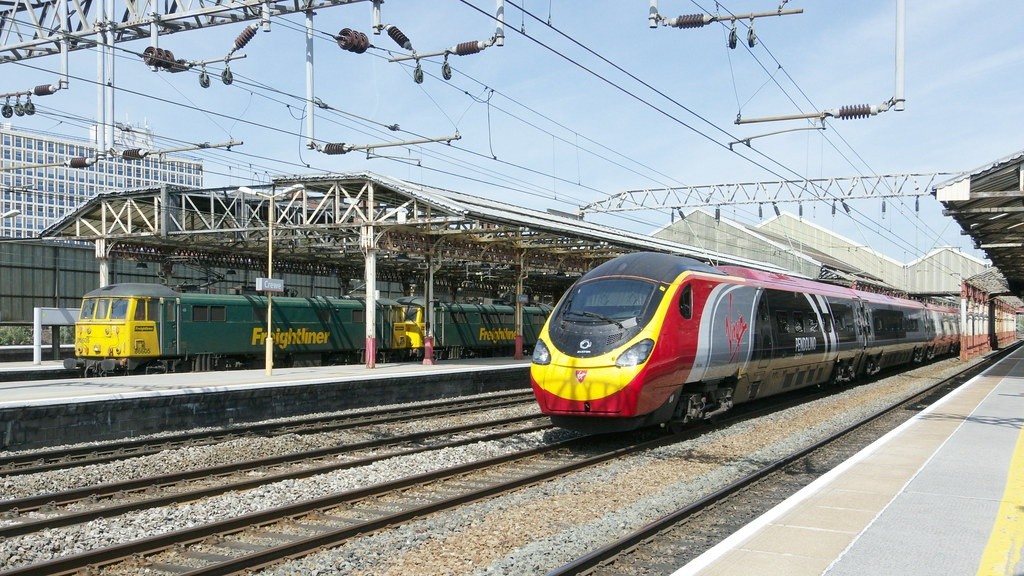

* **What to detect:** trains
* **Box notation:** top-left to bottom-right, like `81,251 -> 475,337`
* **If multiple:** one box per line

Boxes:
64,282 -> 556,381
528,250 -> 961,437
